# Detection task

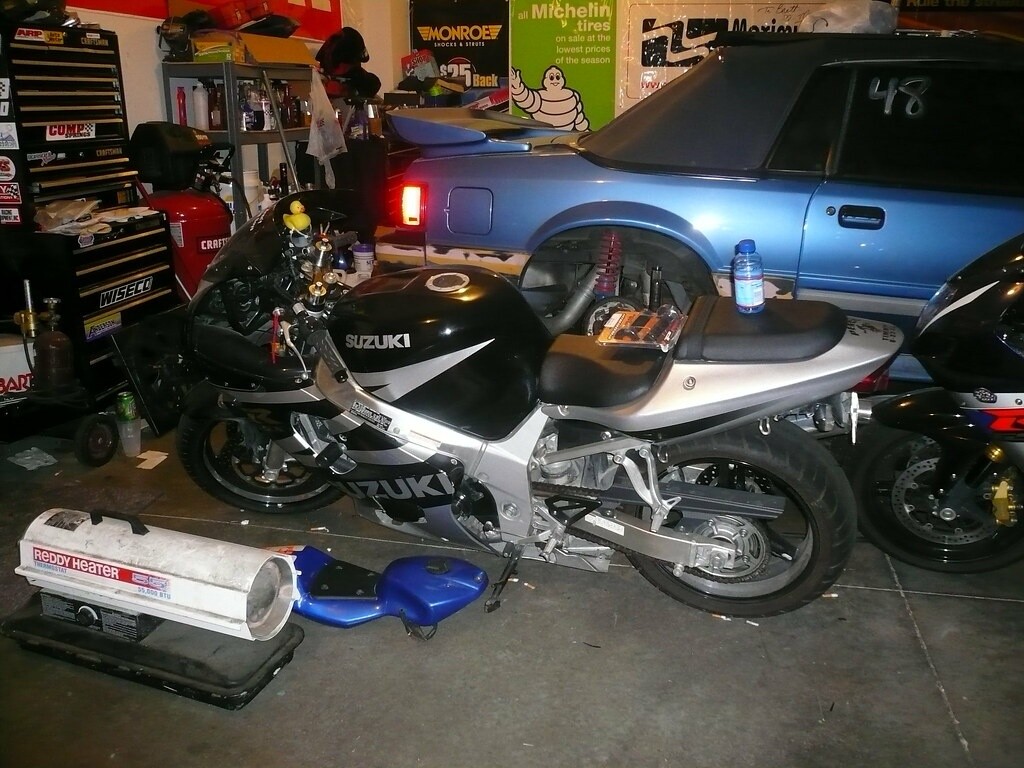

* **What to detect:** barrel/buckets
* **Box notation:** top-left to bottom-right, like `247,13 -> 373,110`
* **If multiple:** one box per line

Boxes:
210,169 -> 262,237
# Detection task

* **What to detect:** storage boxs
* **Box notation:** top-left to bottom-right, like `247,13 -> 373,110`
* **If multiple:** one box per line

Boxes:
191,29 -> 320,66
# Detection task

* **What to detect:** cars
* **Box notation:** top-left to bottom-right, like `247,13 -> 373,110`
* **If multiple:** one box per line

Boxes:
383,28 -> 1024,316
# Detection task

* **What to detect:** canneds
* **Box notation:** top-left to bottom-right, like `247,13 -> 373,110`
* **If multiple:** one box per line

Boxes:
116,393 -> 138,421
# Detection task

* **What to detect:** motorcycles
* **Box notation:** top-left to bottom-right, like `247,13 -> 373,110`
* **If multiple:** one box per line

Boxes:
173,186 -> 906,620
841,232 -> 1023,575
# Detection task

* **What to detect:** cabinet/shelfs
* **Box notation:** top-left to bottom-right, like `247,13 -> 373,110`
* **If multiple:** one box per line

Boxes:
162,60 -> 325,232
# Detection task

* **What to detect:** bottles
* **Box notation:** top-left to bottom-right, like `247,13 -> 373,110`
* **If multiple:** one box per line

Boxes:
192,82 -> 209,131
733,239 -> 764,314
261,96 -> 271,130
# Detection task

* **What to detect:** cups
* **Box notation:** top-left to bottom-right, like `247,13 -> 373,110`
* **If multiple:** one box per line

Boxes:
352,244 -> 374,273
114,413 -> 141,457
